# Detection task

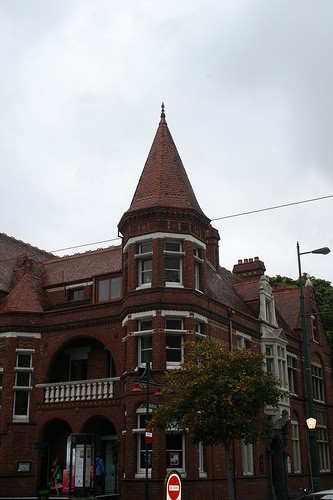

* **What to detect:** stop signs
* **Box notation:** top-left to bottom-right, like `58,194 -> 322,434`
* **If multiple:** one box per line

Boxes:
145,427 -> 152,444
163,470 -> 183,500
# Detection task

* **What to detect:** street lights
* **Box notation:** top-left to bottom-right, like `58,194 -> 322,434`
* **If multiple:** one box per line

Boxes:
131,358 -> 162,500
296,242 -> 329,493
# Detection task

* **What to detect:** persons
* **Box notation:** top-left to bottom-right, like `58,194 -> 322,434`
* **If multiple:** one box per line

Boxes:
94,452 -> 106,496
51,455 -> 62,495
